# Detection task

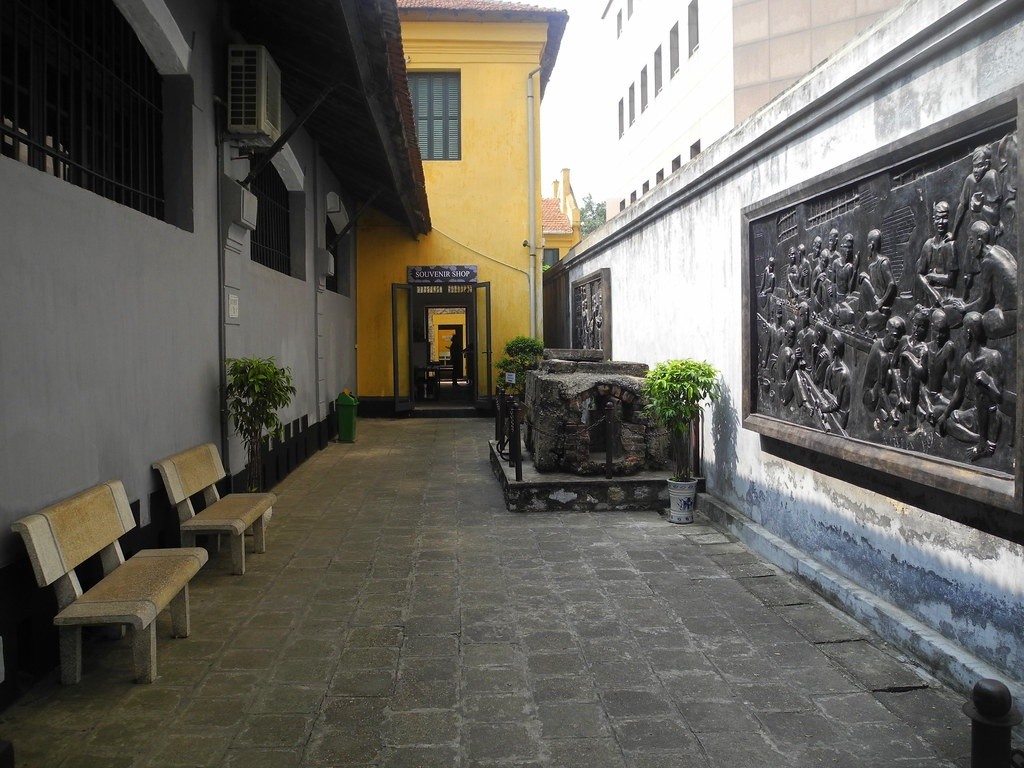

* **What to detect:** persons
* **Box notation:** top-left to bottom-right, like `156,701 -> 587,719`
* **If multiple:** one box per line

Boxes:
571,278 -> 604,350
750,138 -> 1023,467
444,334 -> 475,389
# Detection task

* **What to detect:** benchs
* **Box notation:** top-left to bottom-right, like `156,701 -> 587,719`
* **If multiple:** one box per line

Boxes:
10,478 -> 209,686
151,442 -> 278,575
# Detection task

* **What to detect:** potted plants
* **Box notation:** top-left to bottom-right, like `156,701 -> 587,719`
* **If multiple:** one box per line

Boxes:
642,357 -> 725,524
222,354 -> 297,537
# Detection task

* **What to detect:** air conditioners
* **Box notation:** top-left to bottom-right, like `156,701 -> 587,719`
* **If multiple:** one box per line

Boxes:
225,40 -> 283,150
